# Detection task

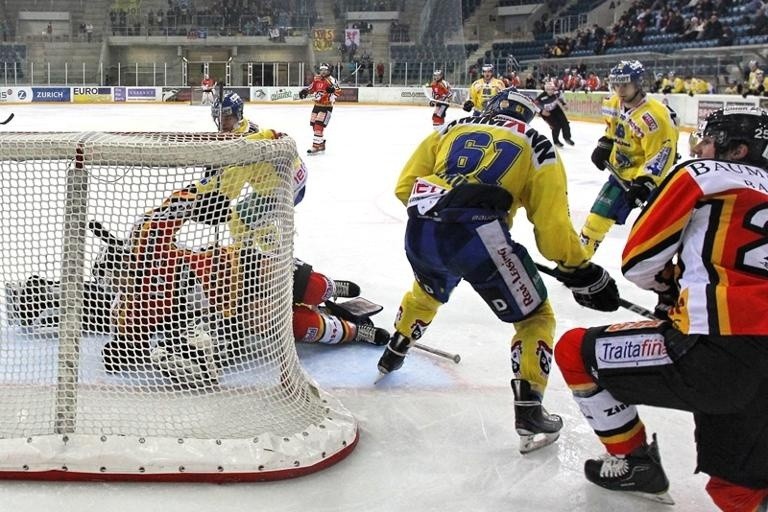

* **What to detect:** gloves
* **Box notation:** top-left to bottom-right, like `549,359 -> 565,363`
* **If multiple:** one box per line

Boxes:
623,176 -> 658,209
299,89 -> 309,99
556,261 -> 619,311
326,86 -> 335,93
591,136 -> 613,171
463,100 -> 474,112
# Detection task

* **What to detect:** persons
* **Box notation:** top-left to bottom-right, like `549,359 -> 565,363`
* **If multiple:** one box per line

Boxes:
469,45 -> 492,74
376,84 -> 620,454
576,60 -> 678,265
424,69 -> 453,128
38,0 -> 319,42
296,62 -> 339,154
332,0 -> 409,83
534,80 -> 577,148
3,88 -> 394,384
254,79 -> 261,85
200,71 -> 220,109
553,108 -> 767,511
461,63 -> 507,119
500,2 -> 767,100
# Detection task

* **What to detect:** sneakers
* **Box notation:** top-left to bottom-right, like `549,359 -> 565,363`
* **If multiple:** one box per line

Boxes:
319,140 -> 326,150
510,379 -> 563,435
20,276 -> 59,325
553,136 -> 564,146
352,323 -> 390,347
564,136 -> 574,145
307,135 -> 321,153
332,280 -> 360,298
377,332 -> 410,374
585,432 -> 670,495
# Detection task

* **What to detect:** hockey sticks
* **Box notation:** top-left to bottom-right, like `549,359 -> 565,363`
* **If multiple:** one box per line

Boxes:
0,112 -> 15,125
89,218 -> 463,363
312,65 -> 363,103
421,84 -> 463,109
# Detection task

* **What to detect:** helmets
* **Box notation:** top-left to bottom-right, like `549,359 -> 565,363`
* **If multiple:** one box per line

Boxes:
211,92 -> 243,132
318,63 -> 333,78
481,64 -> 495,75
607,58 -> 645,103
433,70 -> 444,82
481,86 -> 541,122
544,82 -> 555,91
688,104 -> 768,160
